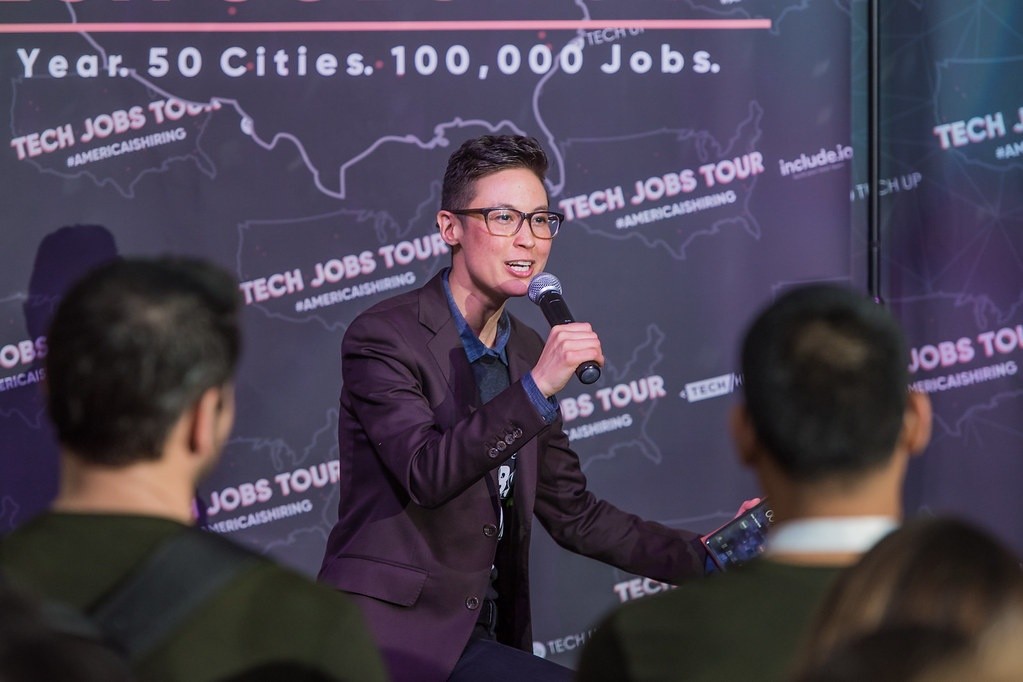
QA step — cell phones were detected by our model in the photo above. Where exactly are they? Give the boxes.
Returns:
[701,498,773,574]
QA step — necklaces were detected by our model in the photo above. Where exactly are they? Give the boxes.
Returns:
[763,515,898,557]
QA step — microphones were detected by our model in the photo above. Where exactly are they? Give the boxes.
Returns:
[528,272,601,385]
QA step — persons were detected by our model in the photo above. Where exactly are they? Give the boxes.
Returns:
[787,511,1023,682]
[0,261,389,682]
[315,133,762,682]
[577,282,931,682]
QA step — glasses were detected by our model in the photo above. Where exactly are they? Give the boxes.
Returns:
[435,207,565,239]
